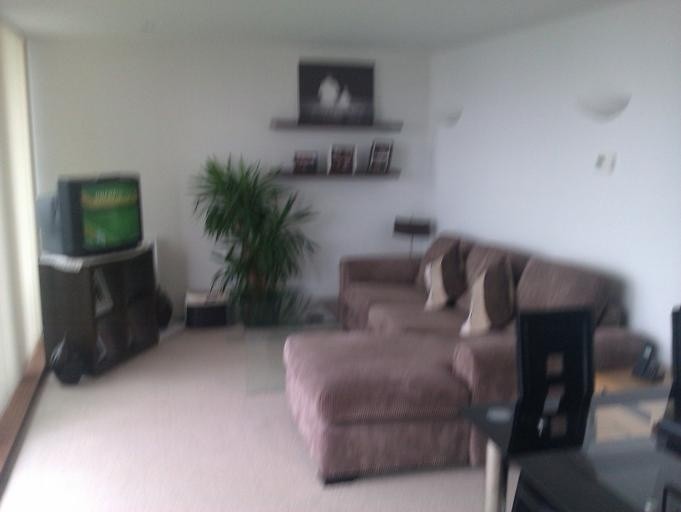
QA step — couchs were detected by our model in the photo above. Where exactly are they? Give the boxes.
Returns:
[341,235,652,470]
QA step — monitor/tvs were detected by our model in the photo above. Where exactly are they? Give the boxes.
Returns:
[35,172,143,255]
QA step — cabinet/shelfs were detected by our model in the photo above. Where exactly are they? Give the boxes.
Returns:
[39,236,161,378]
[269,118,404,179]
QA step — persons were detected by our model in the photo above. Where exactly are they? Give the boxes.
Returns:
[317,72,340,109]
[336,82,352,110]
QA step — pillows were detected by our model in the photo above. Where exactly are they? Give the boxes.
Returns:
[423,239,518,338]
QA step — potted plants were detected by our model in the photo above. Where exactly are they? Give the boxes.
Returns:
[190,147,322,328]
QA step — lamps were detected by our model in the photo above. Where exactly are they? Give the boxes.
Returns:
[393,215,432,258]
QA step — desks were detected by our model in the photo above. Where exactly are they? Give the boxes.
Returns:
[456,385,681,511]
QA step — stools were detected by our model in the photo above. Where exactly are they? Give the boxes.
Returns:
[282,331,473,486]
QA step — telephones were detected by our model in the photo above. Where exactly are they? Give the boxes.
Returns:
[634,342,661,380]
